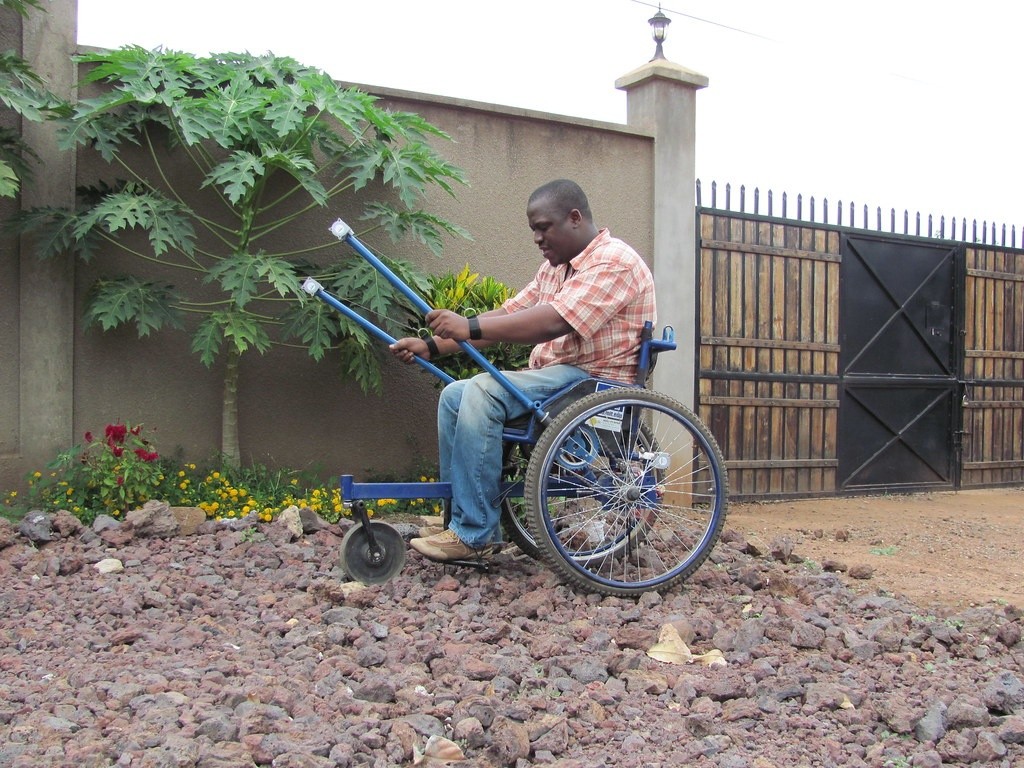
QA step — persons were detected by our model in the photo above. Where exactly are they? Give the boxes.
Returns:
[389,179,658,562]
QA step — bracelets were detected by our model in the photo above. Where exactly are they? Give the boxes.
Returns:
[416,328,441,362]
[464,307,482,342]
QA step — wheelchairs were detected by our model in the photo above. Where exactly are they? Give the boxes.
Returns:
[299,218,731,599]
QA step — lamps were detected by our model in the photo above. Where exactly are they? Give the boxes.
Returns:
[648,3,671,63]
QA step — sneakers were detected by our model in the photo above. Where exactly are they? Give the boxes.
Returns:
[419,526,502,554]
[410,528,493,562]
